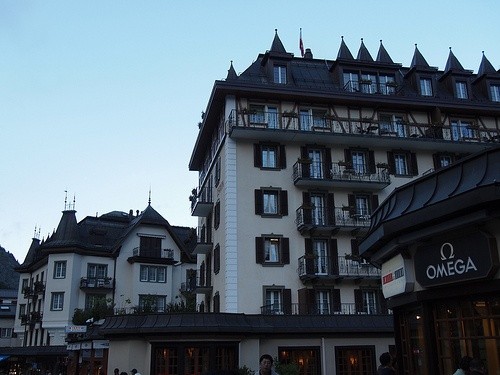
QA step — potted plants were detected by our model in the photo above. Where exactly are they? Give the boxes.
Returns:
[345,252,358,260]
[363,116,374,123]
[385,81,399,87]
[304,251,319,260]
[376,163,386,168]
[187,268,195,279]
[186,228,196,240]
[282,110,298,118]
[338,159,353,166]
[341,204,356,211]
[297,156,313,164]
[432,119,445,125]
[467,123,482,129]
[324,113,336,121]
[396,119,406,125]
[358,78,372,85]
[243,106,257,115]
[301,201,316,210]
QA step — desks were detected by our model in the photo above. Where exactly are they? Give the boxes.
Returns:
[351,213,363,224]
[340,169,353,179]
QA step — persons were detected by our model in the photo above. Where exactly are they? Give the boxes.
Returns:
[254,354,279,375]
[131,369,141,375]
[452,356,488,375]
[114,368,120,375]
[378,349,400,375]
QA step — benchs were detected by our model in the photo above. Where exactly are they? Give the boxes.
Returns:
[310,125,331,132]
[250,121,268,128]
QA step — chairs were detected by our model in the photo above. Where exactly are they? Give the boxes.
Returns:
[330,169,339,178]
